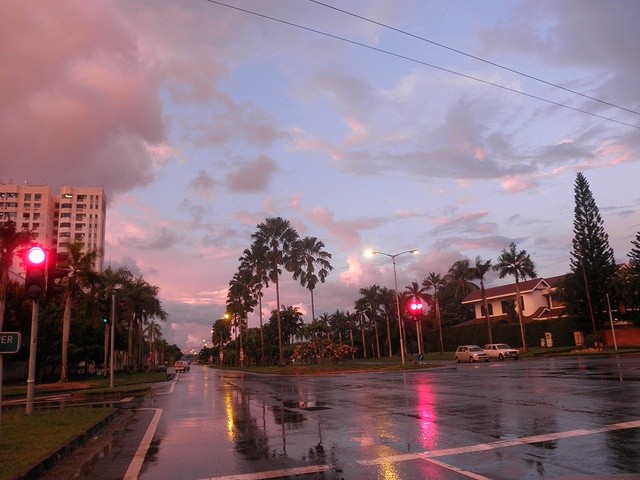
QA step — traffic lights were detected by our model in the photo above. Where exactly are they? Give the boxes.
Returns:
[46,251,70,298]
[98,296,112,324]
[25,242,45,300]
[115,293,127,327]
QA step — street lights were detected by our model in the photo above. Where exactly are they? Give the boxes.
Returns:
[224,315,244,368]
[363,249,420,367]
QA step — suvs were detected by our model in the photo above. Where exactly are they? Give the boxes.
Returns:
[174,361,191,372]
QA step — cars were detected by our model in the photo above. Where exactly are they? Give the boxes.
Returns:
[483,343,520,362]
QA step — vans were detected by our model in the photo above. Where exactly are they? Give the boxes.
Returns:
[455,345,489,363]
